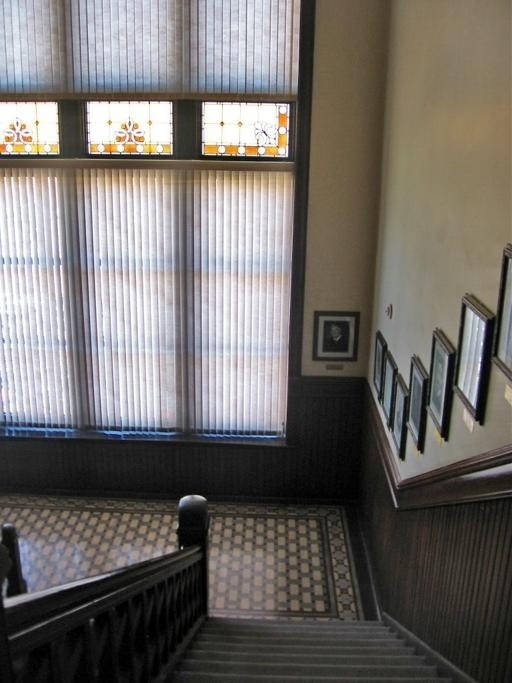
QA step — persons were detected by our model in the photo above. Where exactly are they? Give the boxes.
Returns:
[325,323,347,352]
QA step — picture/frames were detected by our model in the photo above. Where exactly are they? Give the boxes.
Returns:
[370,241,512,460]
[311,310,361,363]
